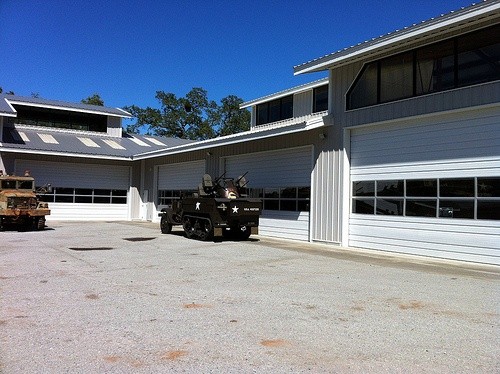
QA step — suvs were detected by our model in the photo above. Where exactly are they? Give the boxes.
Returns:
[0,168,51,230]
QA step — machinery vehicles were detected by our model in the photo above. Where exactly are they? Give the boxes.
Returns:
[157,169,262,242]
